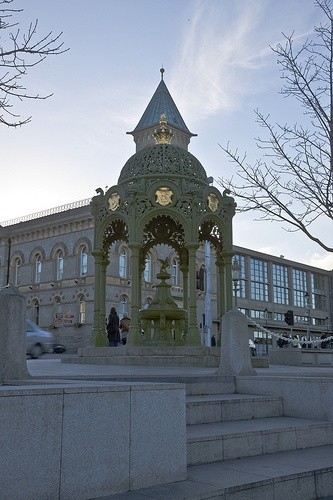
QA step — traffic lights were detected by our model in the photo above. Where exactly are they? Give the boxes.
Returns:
[283,310,292,326]
[196,267,204,292]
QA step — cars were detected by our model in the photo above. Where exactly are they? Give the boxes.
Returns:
[26,319,53,360]
[249,338,257,357]
[47,343,66,355]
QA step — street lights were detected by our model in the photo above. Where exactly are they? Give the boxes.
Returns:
[232,256,242,310]
[263,307,269,355]
[304,292,311,348]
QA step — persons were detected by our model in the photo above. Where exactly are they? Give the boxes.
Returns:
[249,331,333,356]
[105,307,130,347]
[211,333,216,346]
